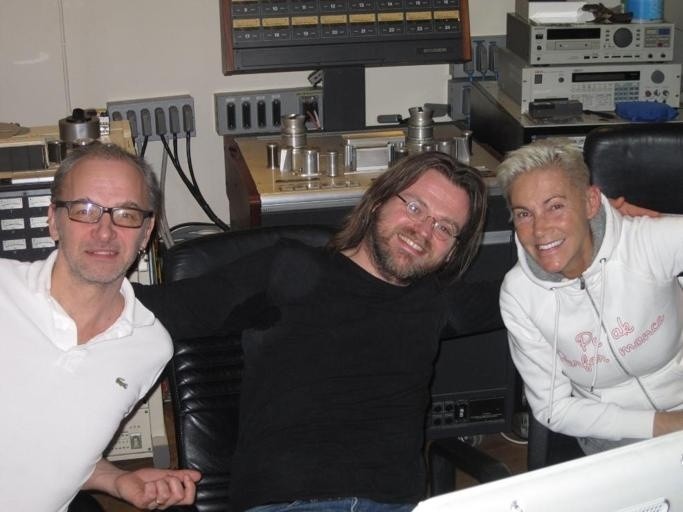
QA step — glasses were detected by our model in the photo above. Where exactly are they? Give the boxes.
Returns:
[396,194,461,241]
[56,200,153,229]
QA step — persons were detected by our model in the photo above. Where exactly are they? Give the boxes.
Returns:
[497,139,683,458]
[131,151,489,511]
[0,139,204,510]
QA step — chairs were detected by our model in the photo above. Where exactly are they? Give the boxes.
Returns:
[163,222,431,512]
[527,122,683,471]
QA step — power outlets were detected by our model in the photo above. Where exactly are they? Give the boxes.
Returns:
[450,35,508,78]
[107,94,197,142]
[212,87,323,135]
[448,81,474,120]
